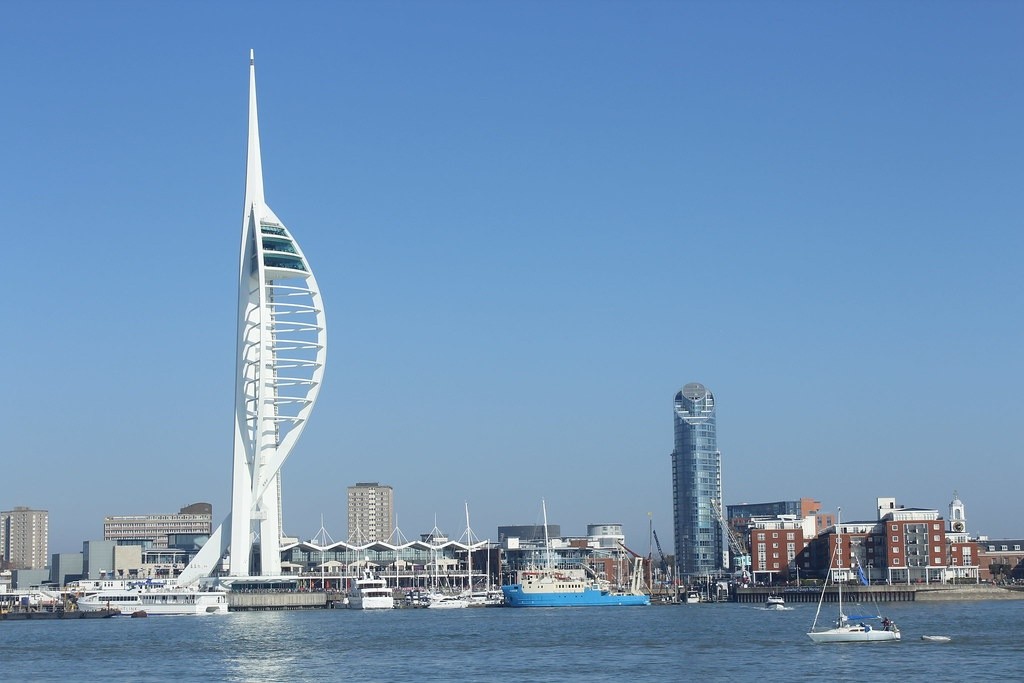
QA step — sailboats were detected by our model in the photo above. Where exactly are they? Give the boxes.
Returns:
[806,505,904,642]
[500,500,651,607]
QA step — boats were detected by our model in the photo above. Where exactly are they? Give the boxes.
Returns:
[324,558,501,609]
[766,596,786,606]
[75,584,230,617]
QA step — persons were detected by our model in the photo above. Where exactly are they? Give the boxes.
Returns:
[881,616,891,630]
